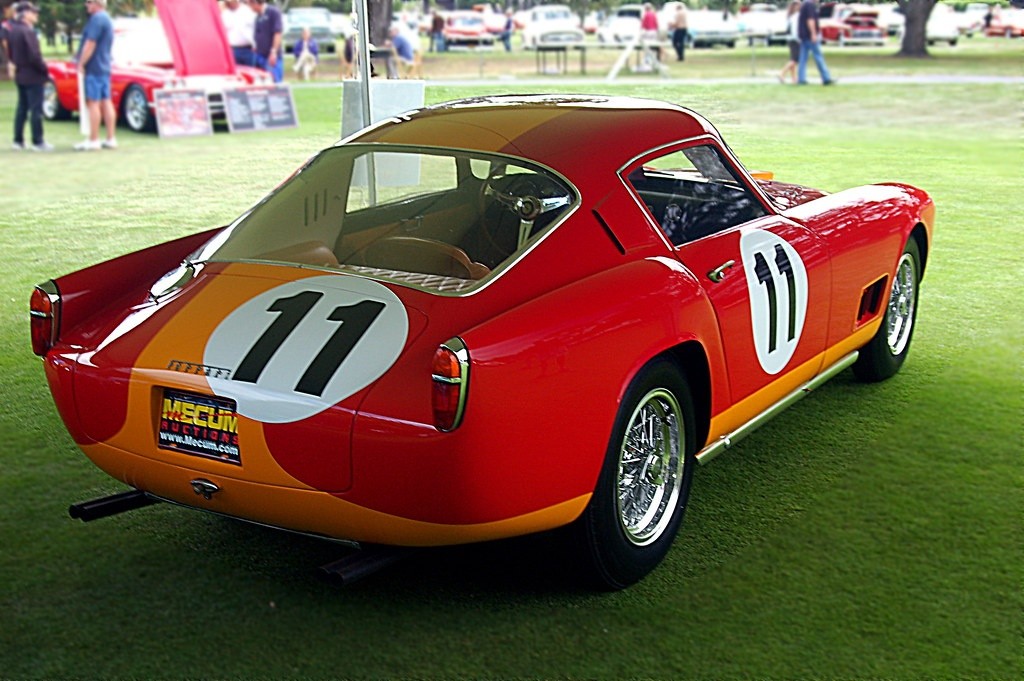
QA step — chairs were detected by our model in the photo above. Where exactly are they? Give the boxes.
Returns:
[337,51,424,82]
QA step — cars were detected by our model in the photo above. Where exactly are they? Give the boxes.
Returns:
[740,2,907,47]
[685,10,740,49]
[600,5,667,50]
[923,1,959,47]
[524,5,585,50]
[44,0,275,134]
[284,5,507,56]
[954,3,1024,40]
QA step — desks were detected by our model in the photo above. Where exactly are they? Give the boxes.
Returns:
[536,43,661,73]
[368,47,393,79]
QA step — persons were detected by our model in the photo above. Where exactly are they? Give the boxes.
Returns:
[641,4,658,39]
[221,0,284,82]
[0,0,54,152]
[797,0,833,85]
[345,37,378,77]
[293,28,318,80]
[502,10,512,51]
[429,10,444,51]
[385,27,414,79]
[669,5,688,60]
[779,0,802,83]
[73,0,117,149]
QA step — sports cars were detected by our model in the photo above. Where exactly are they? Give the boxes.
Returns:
[29,93,937,594]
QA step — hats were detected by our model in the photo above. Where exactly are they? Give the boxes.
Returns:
[15,2,41,14]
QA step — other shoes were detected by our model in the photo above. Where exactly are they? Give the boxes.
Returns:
[73,138,101,150]
[11,142,24,151]
[33,142,52,152]
[100,139,116,151]
